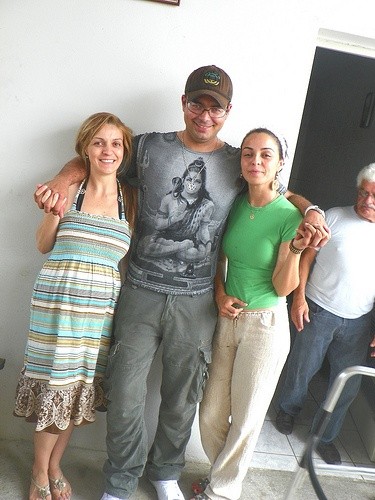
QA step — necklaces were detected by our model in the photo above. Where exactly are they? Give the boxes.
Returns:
[247,190,277,220]
[181,131,219,191]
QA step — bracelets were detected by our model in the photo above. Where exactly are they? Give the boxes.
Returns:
[289,238,303,254]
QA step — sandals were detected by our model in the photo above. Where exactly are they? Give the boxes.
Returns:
[28,470,51,500]
[48,471,72,500]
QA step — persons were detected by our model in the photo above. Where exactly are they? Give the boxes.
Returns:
[190,125,331,500]
[13,112,139,500]
[274,161,375,464]
[34,66,326,500]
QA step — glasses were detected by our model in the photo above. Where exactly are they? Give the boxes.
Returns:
[358,189,375,204]
[187,99,226,119]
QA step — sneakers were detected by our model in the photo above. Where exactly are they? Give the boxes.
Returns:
[99,492,125,500]
[147,477,186,499]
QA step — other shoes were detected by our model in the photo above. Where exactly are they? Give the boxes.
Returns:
[275,409,295,435]
[315,440,342,465]
[188,475,215,500]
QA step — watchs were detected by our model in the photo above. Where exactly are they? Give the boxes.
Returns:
[304,205,325,219]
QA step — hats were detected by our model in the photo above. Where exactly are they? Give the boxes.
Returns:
[185,64,234,110]
